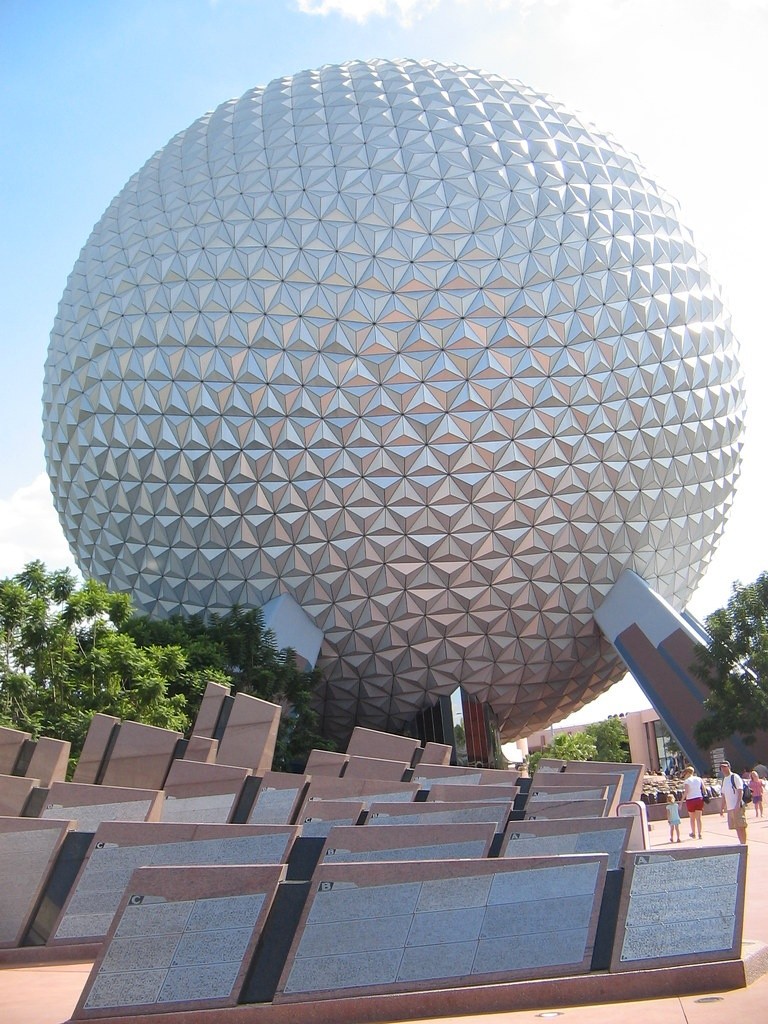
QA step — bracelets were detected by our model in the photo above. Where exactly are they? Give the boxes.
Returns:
[721,807,724,809]
[681,800,684,803]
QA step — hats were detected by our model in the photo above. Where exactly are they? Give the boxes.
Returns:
[719,760,730,767]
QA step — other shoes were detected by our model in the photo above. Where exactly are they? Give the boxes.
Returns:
[688,832,696,838]
[698,835,704,838]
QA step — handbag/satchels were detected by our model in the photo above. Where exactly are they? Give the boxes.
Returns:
[731,772,754,803]
[701,790,711,804]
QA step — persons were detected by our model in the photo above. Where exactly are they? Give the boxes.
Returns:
[660,766,675,775]
[666,794,683,843]
[742,760,768,779]
[720,761,750,855]
[748,771,766,818]
[679,767,706,840]
[686,763,694,772]
